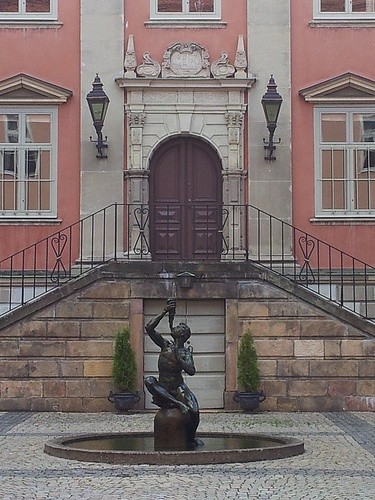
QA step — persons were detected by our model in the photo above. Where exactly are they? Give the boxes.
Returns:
[145,297,204,447]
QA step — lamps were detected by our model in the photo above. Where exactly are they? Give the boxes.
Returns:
[261,74,283,160]
[86,73,110,159]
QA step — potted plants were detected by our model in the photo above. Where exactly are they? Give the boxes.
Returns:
[108,328,141,414]
[232,328,266,414]
